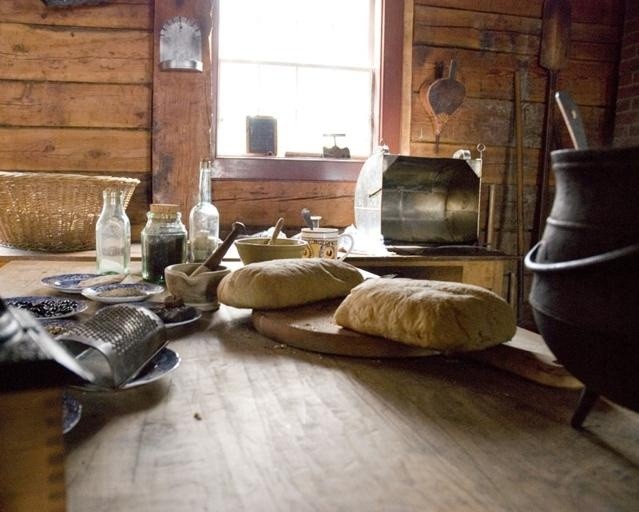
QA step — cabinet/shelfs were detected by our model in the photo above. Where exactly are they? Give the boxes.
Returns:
[343,250,521,296]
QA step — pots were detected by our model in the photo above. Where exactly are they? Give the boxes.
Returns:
[523,146,639,430]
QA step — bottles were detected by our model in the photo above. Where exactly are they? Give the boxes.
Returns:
[95,191,131,276]
[188,157,222,262]
[139,201,189,284]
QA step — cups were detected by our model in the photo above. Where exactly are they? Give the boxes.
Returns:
[300,226,354,263]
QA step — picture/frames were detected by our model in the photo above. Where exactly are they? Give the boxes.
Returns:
[244,115,281,157]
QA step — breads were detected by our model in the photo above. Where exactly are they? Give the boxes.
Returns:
[334,278,517,350]
[217,258,364,309]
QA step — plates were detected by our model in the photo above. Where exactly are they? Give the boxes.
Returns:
[1,296,89,321]
[33,320,182,435]
[40,273,115,293]
[94,301,204,328]
[80,282,165,304]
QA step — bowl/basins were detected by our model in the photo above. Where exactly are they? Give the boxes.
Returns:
[164,262,231,311]
[233,237,308,268]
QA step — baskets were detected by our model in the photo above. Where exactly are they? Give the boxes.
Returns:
[0,170,140,253]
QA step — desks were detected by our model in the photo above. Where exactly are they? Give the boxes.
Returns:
[0,258,637,511]
